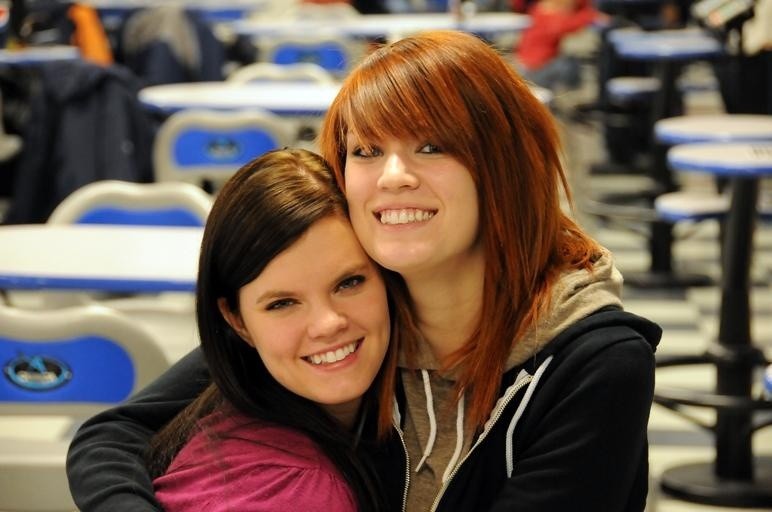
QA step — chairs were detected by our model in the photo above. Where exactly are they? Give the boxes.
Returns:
[1,305,175,510]
[43,177,212,225]
[150,103,295,194]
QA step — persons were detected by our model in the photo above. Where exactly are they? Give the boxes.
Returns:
[63,29,663,511]
[0,0,609,95]
[138,145,425,512]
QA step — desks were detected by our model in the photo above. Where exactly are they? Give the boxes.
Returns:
[137,76,337,156]
[0,220,208,293]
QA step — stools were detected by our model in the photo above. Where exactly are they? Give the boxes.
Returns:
[575,11,772,509]
